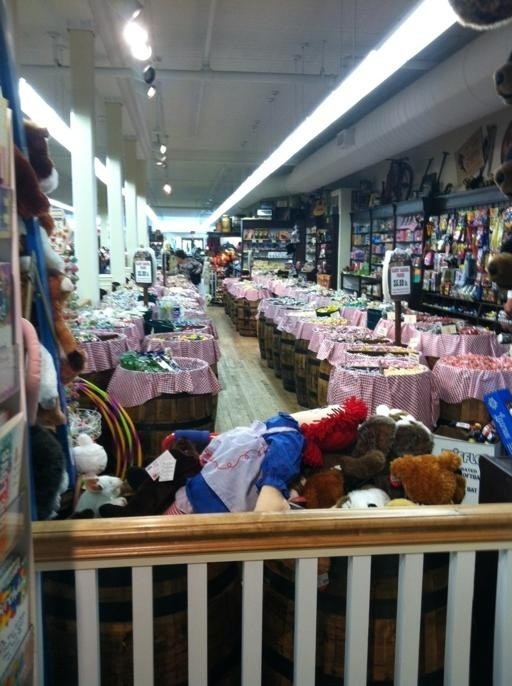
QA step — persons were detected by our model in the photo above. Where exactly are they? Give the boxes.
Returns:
[161,251,207,310]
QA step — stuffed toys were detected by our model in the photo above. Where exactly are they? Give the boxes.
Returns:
[13,123,84,429]
[69,398,467,614]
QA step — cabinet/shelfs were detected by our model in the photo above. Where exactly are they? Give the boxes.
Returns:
[420,184,511,336]
[341,198,438,311]
[241,217,335,287]
